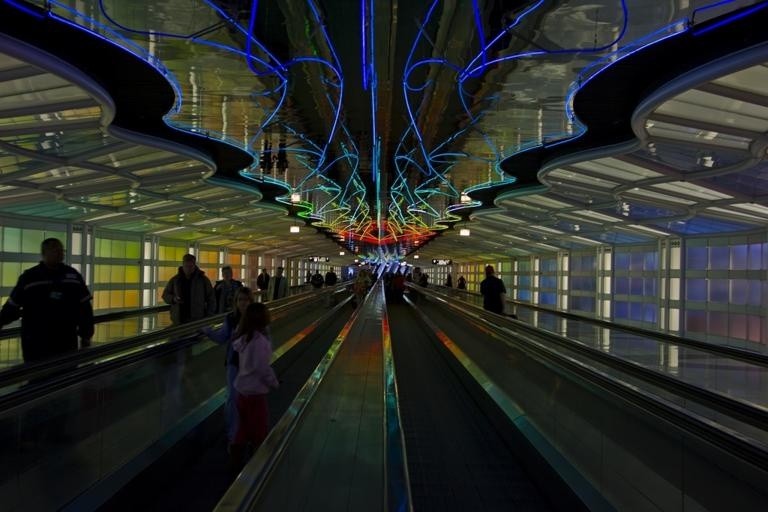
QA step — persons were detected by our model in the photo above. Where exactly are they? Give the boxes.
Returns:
[161,255,216,323]
[446,273,451,287]
[324,267,336,288]
[0,237,95,448]
[256,268,270,301]
[260,138,291,176]
[310,269,323,289]
[212,266,242,313]
[479,266,506,314]
[457,275,466,288]
[353,269,429,303]
[151,343,192,429]
[305,271,311,281]
[230,302,279,448]
[266,267,288,301]
[201,287,274,437]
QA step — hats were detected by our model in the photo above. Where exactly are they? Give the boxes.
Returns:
[486,265,494,274]
